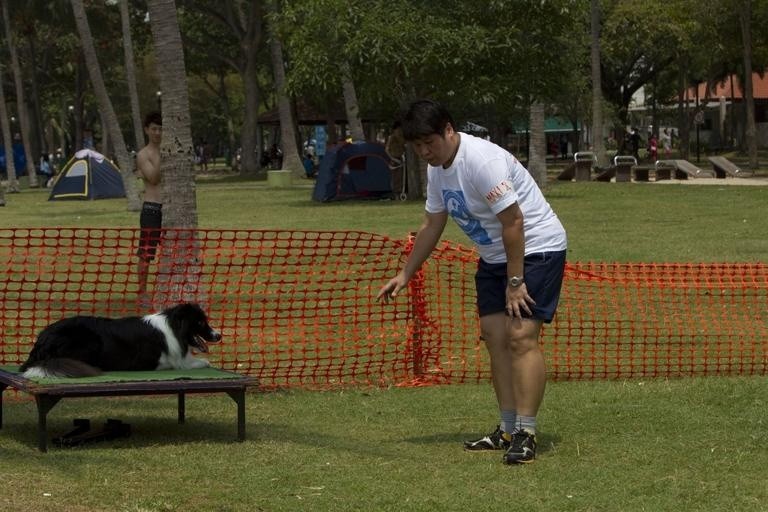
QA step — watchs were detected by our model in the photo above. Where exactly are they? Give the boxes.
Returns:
[506,276,524,287]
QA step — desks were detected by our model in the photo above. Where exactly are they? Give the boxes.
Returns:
[1,362,257,454]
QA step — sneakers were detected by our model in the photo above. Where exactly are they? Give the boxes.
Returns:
[500,426,538,466]
[462,423,512,454]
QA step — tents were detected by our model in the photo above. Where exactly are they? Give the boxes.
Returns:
[313,139,405,203]
[47,148,128,200]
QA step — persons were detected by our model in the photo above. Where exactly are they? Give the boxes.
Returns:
[231,144,284,171]
[303,154,316,178]
[626,129,645,164]
[304,135,317,155]
[560,135,567,160]
[560,141,560,163]
[663,137,671,160]
[374,99,568,465]
[40,152,54,186]
[136,112,162,303]
[196,145,208,174]
[647,134,658,163]
[663,129,672,148]
[385,124,407,201]
[677,129,690,160]
[53,148,66,175]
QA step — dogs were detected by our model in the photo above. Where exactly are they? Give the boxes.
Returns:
[18,303,222,378]
